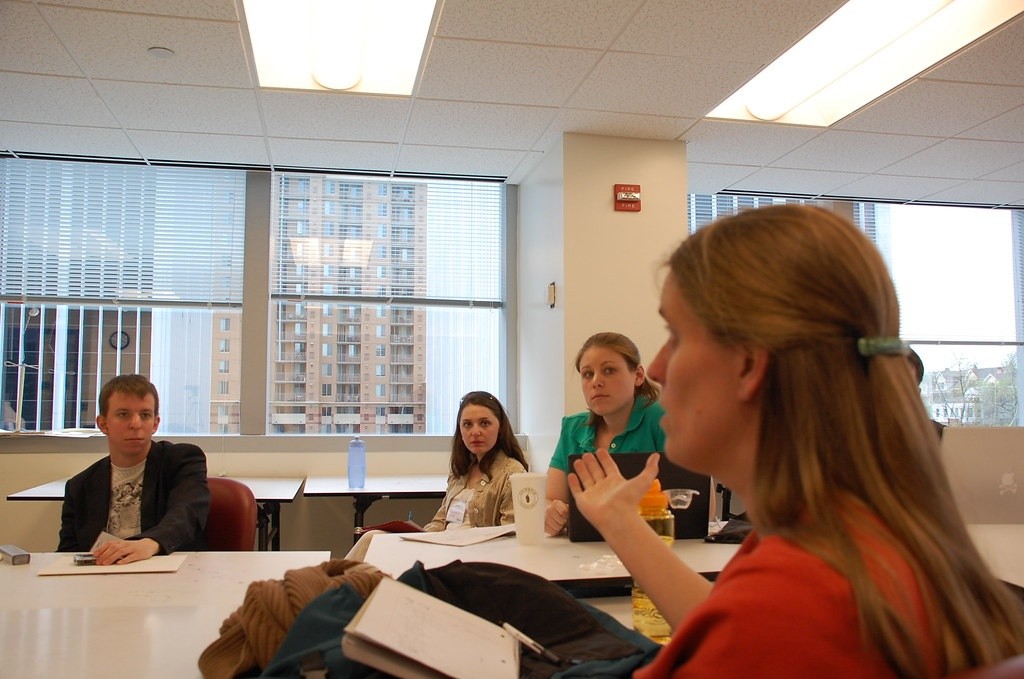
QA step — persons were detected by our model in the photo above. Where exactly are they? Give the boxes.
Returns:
[567,203,1024,679]
[423,391,529,532]
[545,332,716,536]
[57,373,210,566]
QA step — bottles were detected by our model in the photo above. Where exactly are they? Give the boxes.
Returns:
[347,436,367,489]
[631,478,677,646]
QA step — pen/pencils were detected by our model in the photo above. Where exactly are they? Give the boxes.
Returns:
[497,620,562,666]
[408,511,412,521]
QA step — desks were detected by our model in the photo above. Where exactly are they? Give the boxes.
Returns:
[0,550,333,679]
[303,473,461,549]
[7,475,303,551]
[362,534,745,630]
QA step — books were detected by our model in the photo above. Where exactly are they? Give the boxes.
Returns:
[342,576,521,679]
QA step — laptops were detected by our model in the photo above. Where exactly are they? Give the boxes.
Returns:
[567,453,710,543]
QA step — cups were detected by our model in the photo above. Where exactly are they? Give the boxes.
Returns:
[509,473,548,545]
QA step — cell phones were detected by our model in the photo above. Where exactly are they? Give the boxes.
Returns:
[74,553,125,566]
